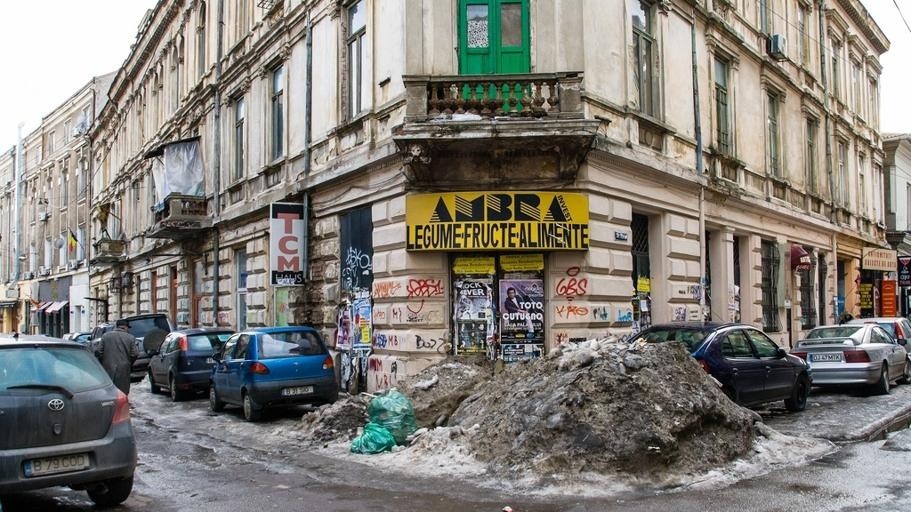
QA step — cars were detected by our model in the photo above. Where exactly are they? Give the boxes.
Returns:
[206,327,339,420]
[627,322,813,412]
[789,323,910,393]
[848,316,911,353]
[144,328,239,403]
[0,331,138,510]
[69,331,91,344]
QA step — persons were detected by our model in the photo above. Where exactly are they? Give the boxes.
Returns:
[505,287,528,326]
[98,318,140,398]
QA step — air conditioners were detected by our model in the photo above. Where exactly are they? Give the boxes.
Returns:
[770,32,791,64]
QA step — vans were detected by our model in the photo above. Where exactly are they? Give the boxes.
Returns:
[112,314,174,380]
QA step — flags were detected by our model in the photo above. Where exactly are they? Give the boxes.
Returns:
[68,228,78,257]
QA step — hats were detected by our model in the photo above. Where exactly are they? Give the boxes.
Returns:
[117,319,132,328]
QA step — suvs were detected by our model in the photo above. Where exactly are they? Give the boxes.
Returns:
[87,322,115,358]
